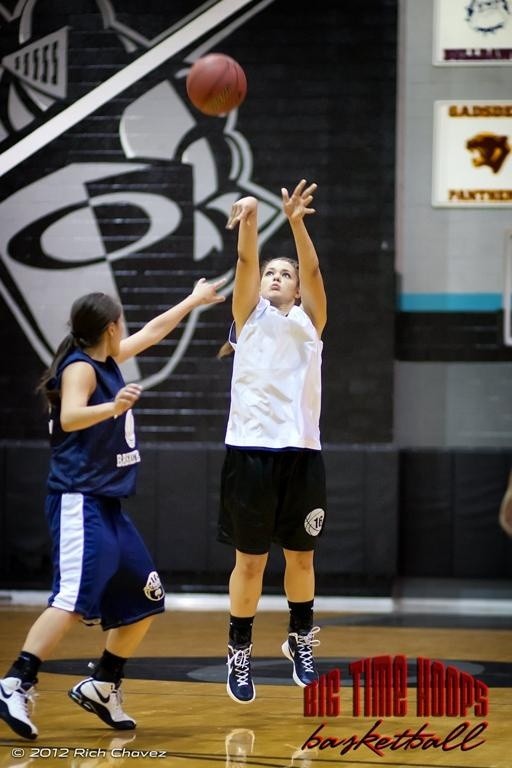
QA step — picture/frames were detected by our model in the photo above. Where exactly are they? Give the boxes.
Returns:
[430,0,511,210]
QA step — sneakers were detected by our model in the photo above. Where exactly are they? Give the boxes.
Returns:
[287,749,318,768]
[225,729,255,768]
[226,640,256,704]
[282,626,320,688]
[68,677,136,730]
[0,677,39,739]
[72,732,136,768]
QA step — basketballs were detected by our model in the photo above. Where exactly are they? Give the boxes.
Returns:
[187,52,248,117]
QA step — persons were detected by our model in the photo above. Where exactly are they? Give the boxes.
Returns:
[213,175,329,706]
[0,272,230,744]
